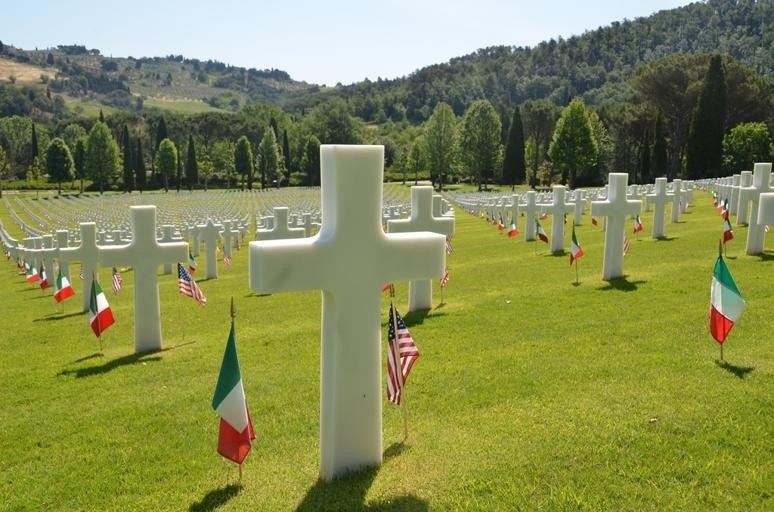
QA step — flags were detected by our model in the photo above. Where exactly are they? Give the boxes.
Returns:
[6,251,123,339]
[386,303,420,407]
[177,262,206,307]
[708,255,747,345]
[712,191,735,244]
[186,240,232,275]
[439,210,644,291]
[209,321,257,465]
